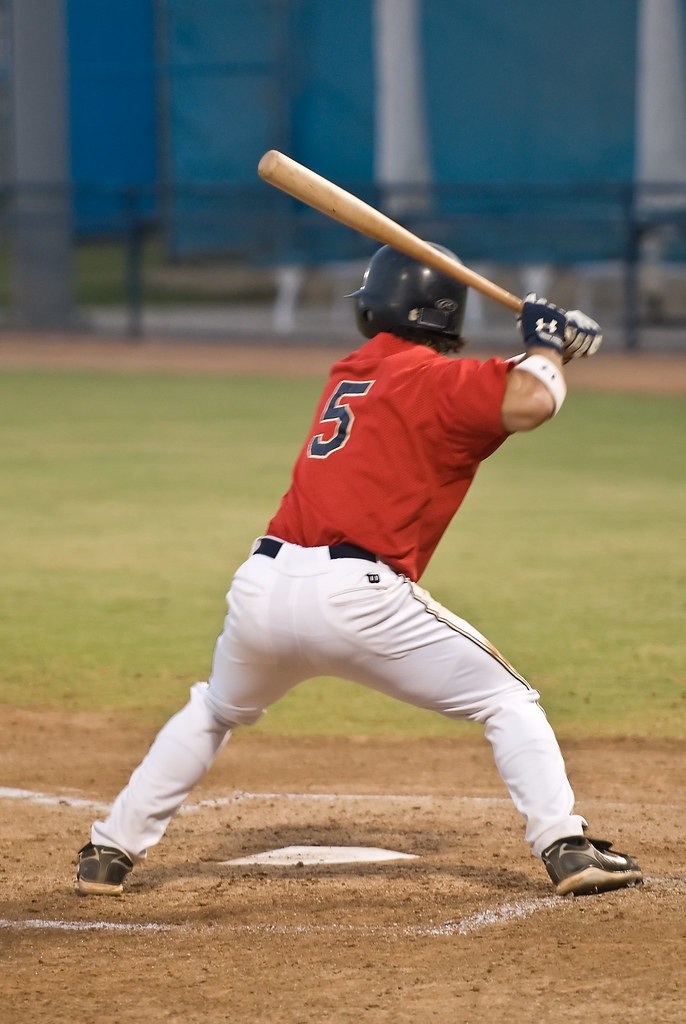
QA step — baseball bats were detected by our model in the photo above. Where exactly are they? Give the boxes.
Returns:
[256,149,525,312]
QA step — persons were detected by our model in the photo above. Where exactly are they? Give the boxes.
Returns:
[74,241,644,896]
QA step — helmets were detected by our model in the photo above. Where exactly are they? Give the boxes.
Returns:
[344,238,467,338]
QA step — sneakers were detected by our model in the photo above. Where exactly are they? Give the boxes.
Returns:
[76,842,134,895]
[540,835,643,895]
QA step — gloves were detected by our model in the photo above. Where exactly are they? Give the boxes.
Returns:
[562,309,602,366]
[515,291,566,357]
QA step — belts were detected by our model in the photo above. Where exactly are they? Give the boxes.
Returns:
[255,536,381,563]
